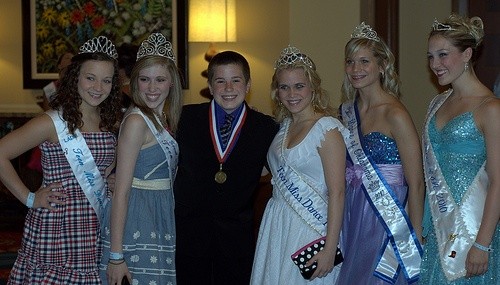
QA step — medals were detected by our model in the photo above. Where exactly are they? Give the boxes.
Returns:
[215,172,226,183]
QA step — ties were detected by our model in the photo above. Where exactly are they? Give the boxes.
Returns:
[219,114,235,148]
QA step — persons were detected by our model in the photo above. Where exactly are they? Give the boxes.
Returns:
[108,52,282,285]
[336,21,426,285]
[105,33,180,285]
[419,14,500,285]
[249,43,344,285]
[0,36,117,285]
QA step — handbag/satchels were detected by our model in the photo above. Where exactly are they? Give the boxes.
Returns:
[291,234,345,280]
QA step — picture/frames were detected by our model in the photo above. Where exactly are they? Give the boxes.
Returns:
[21,0,190,92]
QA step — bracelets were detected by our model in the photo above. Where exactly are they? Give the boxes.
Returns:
[26,191,35,209]
[107,258,125,265]
[108,251,124,260]
[473,241,492,253]
